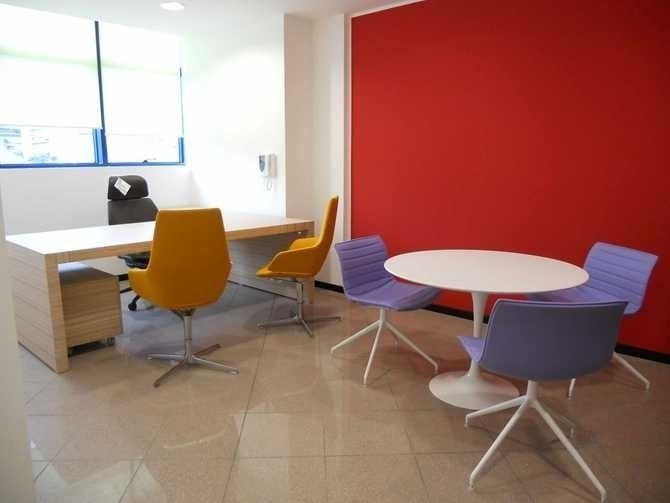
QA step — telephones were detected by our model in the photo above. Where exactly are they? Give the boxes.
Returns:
[258,153,277,177]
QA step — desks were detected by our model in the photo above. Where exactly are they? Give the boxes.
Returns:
[382,249,589,411]
[5,213,316,373]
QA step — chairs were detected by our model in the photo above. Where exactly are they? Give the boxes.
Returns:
[331,235,442,386]
[529,241,659,400]
[107,174,159,310]
[127,209,238,388]
[255,195,341,337]
[455,299,629,503]
